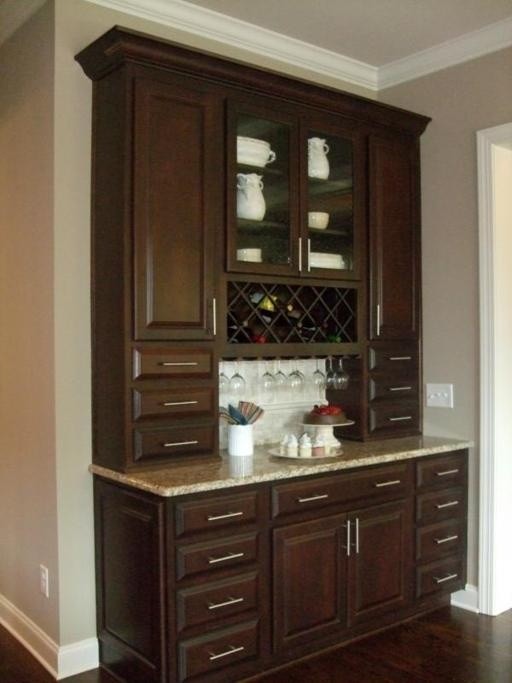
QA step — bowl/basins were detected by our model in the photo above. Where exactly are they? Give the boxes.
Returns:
[308,211,331,230]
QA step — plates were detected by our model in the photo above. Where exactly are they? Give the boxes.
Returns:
[289,420,358,447]
[267,447,346,461]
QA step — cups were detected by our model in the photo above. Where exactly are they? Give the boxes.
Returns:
[236,247,264,263]
[271,359,288,391]
[324,355,343,389]
[217,361,232,395]
[259,361,277,392]
[310,357,325,390]
[285,358,304,393]
[332,356,350,392]
[229,361,247,398]
[229,423,254,458]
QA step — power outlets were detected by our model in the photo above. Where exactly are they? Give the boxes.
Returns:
[39,563,49,600]
[426,383,454,409]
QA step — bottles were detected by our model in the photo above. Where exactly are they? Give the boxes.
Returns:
[250,293,293,314]
[230,329,265,344]
[255,311,302,328]
[324,335,342,343]
[296,320,329,337]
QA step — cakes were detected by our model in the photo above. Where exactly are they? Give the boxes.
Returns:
[287,434,298,456]
[312,436,325,456]
[305,405,347,426]
[280,436,288,453]
[299,432,312,456]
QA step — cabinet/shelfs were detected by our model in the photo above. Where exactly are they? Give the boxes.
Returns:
[86,436,476,683]
[74,26,431,471]
